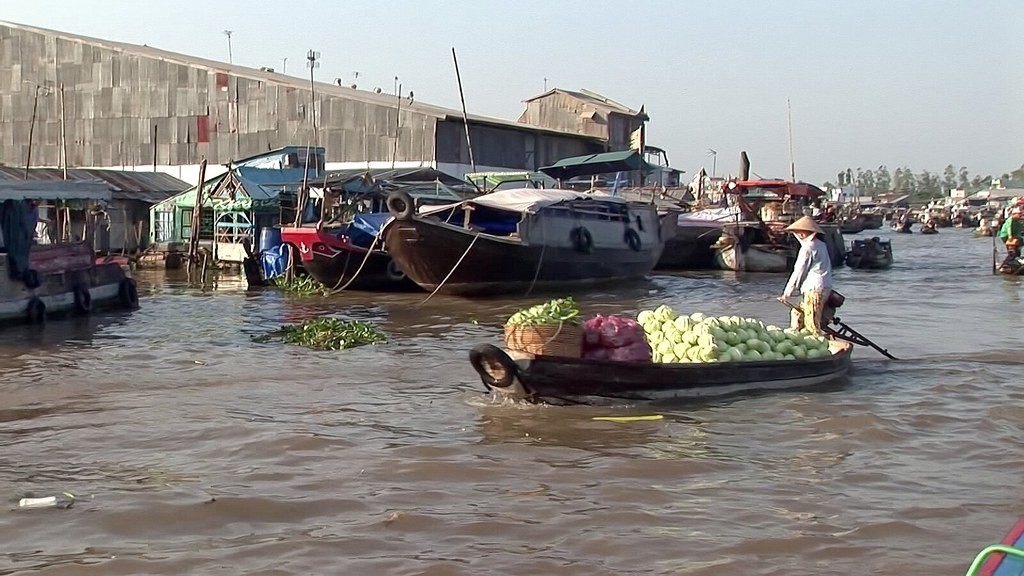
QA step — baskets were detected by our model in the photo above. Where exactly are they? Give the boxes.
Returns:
[504,324,583,360]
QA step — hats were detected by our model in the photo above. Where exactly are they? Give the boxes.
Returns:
[1011,208,1022,214]
[784,215,826,234]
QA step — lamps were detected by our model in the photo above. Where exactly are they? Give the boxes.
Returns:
[407,91,414,101]
[373,87,382,94]
[260,65,274,72]
[350,84,357,89]
[333,77,341,87]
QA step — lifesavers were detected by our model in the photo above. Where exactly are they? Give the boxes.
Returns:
[119,278,138,308]
[469,341,517,388]
[72,285,93,312]
[576,226,594,254]
[27,296,46,315]
[387,192,417,217]
[625,228,641,251]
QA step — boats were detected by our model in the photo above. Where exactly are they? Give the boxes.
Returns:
[468,289,853,407]
[278,47,1024,296]
[0,178,136,322]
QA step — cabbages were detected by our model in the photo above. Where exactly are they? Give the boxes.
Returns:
[506,300,576,324]
[637,304,830,364]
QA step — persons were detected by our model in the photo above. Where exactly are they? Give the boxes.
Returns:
[781,215,833,338]
[887,196,1024,267]
[33,200,113,246]
